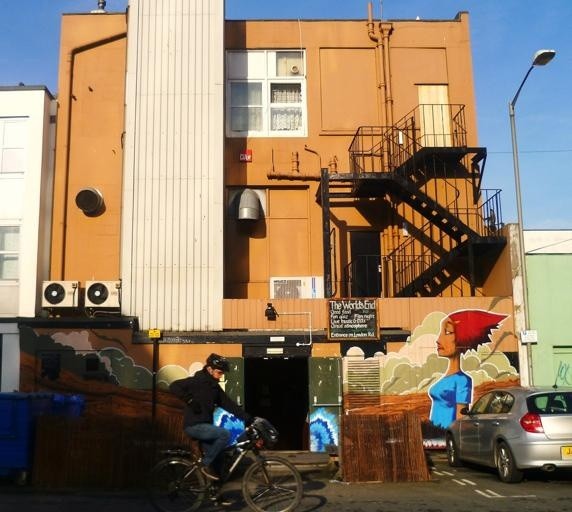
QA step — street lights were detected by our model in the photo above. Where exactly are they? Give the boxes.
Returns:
[510,45,560,386]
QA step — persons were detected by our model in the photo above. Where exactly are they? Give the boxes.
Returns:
[183,353,256,505]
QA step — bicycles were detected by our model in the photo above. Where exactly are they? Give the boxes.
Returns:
[144,417,305,511]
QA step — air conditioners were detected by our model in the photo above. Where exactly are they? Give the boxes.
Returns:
[40,279,121,311]
[269,276,325,300]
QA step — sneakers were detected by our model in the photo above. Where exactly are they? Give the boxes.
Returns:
[202,466,220,481]
[221,498,235,506]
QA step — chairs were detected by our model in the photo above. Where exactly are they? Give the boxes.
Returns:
[547,400,568,412]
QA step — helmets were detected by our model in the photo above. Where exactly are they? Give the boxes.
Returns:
[206,352,231,374]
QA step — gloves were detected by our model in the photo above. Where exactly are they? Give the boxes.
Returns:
[187,399,202,414]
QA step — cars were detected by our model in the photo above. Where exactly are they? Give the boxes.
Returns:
[445,383,572,484]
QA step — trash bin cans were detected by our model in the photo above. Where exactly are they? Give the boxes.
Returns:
[0,391,85,484]
